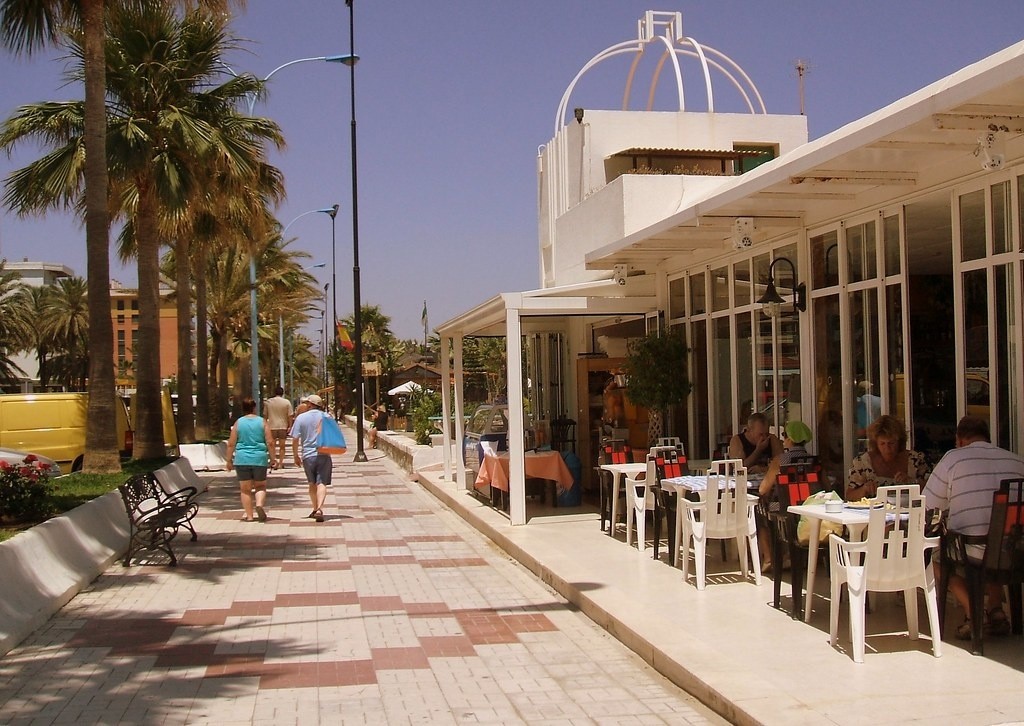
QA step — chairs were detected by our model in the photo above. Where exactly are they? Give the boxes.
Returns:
[656,437,686,457]
[938,477,1024,656]
[697,442,732,547]
[649,449,728,568]
[681,458,762,590]
[624,442,685,552]
[776,472,872,620]
[593,436,645,537]
[759,454,824,574]
[829,485,942,662]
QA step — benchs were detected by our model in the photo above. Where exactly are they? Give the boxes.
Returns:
[118,471,199,568]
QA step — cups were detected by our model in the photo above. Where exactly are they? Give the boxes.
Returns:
[824,501,843,513]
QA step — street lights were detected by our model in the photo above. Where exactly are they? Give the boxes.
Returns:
[278,208,332,397]
[248,53,359,412]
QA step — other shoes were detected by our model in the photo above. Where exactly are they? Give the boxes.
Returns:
[783,559,791,569]
[761,563,771,573]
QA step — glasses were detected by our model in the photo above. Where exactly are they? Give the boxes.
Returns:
[781,432,786,436]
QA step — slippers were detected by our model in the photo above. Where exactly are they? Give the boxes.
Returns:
[240,517,254,522]
[255,506,267,523]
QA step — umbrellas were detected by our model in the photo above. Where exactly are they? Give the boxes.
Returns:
[388,380,434,399]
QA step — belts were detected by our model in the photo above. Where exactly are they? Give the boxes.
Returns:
[961,534,987,544]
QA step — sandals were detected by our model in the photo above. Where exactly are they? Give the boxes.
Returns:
[308,511,316,518]
[983,607,1010,635]
[315,508,324,522]
[954,615,972,640]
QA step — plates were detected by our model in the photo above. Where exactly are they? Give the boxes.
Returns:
[843,503,884,509]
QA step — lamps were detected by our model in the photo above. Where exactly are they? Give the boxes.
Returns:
[574,108,584,123]
[756,257,806,316]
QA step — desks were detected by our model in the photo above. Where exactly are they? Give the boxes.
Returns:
[660,475,762,578]
[474,448,576,512]
[787,490,927,644]
[600,460,712,546]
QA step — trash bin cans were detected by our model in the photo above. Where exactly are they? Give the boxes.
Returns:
[557,449,581,509]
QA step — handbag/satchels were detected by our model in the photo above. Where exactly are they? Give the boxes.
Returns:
[316,412,346,454]
[796,490,843,547]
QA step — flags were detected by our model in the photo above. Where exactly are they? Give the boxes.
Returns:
[421,307,429,325]
[337,322,353,349]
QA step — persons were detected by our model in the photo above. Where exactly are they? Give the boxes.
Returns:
[729,413,784,475]
[846,415,931,501]
[919,416,1024,640]
[756,422,815,572]
[263,387,293,470]
[288,395,332,522]
[294,396,308,417]
[856,380,874,424]
[364,404,387,450]
[226,396,278,522]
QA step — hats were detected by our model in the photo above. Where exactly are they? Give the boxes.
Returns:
[786,420,812,443]
[303,395,322,407]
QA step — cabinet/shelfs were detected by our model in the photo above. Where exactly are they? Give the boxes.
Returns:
[576,356,643,494]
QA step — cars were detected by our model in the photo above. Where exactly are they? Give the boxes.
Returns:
[1,390,197,479]
[764,358,991,440]
